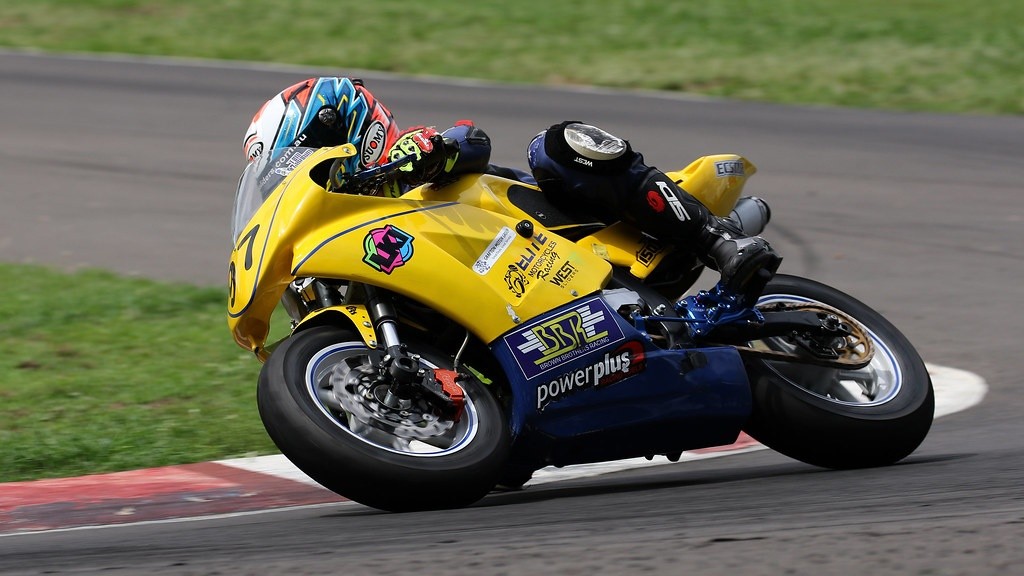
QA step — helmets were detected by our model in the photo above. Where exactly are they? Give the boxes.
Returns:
[242,76,401,198]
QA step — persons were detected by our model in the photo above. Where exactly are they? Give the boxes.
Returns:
[242,76,778,297]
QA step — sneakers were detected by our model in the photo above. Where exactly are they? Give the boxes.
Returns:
[692,215,779,292]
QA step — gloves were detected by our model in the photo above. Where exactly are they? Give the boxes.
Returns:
[385,125,447,180]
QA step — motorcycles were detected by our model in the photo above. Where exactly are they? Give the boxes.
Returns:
[226,139,937,510]
